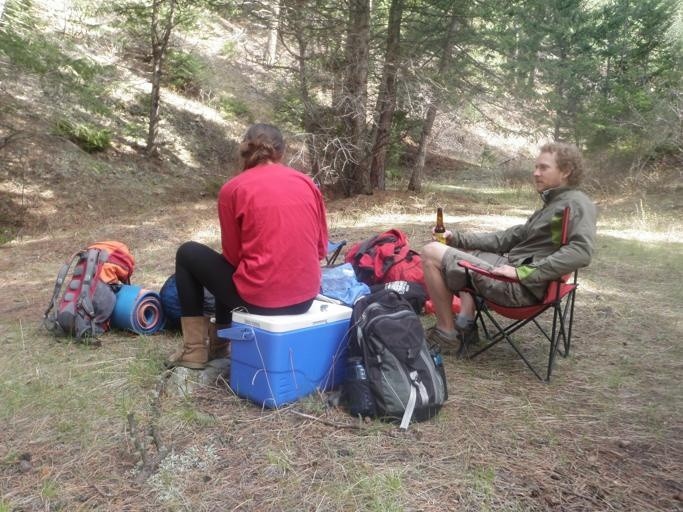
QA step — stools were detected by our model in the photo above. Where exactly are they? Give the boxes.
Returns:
[323,239,347,265]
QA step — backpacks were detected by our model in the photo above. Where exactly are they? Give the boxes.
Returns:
[345,290,449,422]
[45,238,135,343]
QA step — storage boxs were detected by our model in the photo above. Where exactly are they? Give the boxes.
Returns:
[216,298,353,410]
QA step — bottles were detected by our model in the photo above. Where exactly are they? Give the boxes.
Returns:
[345,359,374,417]
[431,350,449,401]
[434,206,447,247]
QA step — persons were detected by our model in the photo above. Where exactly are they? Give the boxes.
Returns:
[164,123,331,371]
[419,141,597,356]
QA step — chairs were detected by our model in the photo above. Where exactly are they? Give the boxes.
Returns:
[455,207,582,382]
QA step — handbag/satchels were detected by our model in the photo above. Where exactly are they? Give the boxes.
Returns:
[346,227,461,313]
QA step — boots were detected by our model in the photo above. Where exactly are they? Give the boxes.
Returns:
[207,322,230,359]
[164,315,209,370]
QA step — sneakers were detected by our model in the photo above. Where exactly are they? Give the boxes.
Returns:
[425,324,464,355]
[454,320,480,345]
